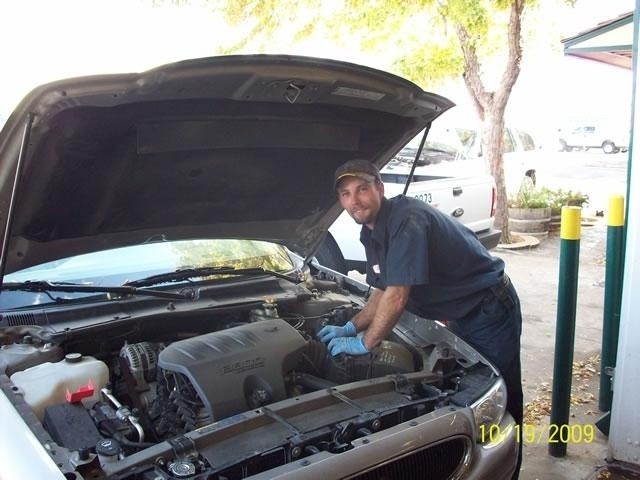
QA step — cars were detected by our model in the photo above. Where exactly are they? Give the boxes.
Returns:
[332,135,501,271]
[555,120,630,155]
[0,53,520,480]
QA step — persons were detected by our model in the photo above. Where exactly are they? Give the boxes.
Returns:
[317,158,524,480]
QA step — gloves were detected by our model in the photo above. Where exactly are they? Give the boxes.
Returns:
[316,321,369,357]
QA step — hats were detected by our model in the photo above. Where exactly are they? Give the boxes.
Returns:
[334,160,381,186]
